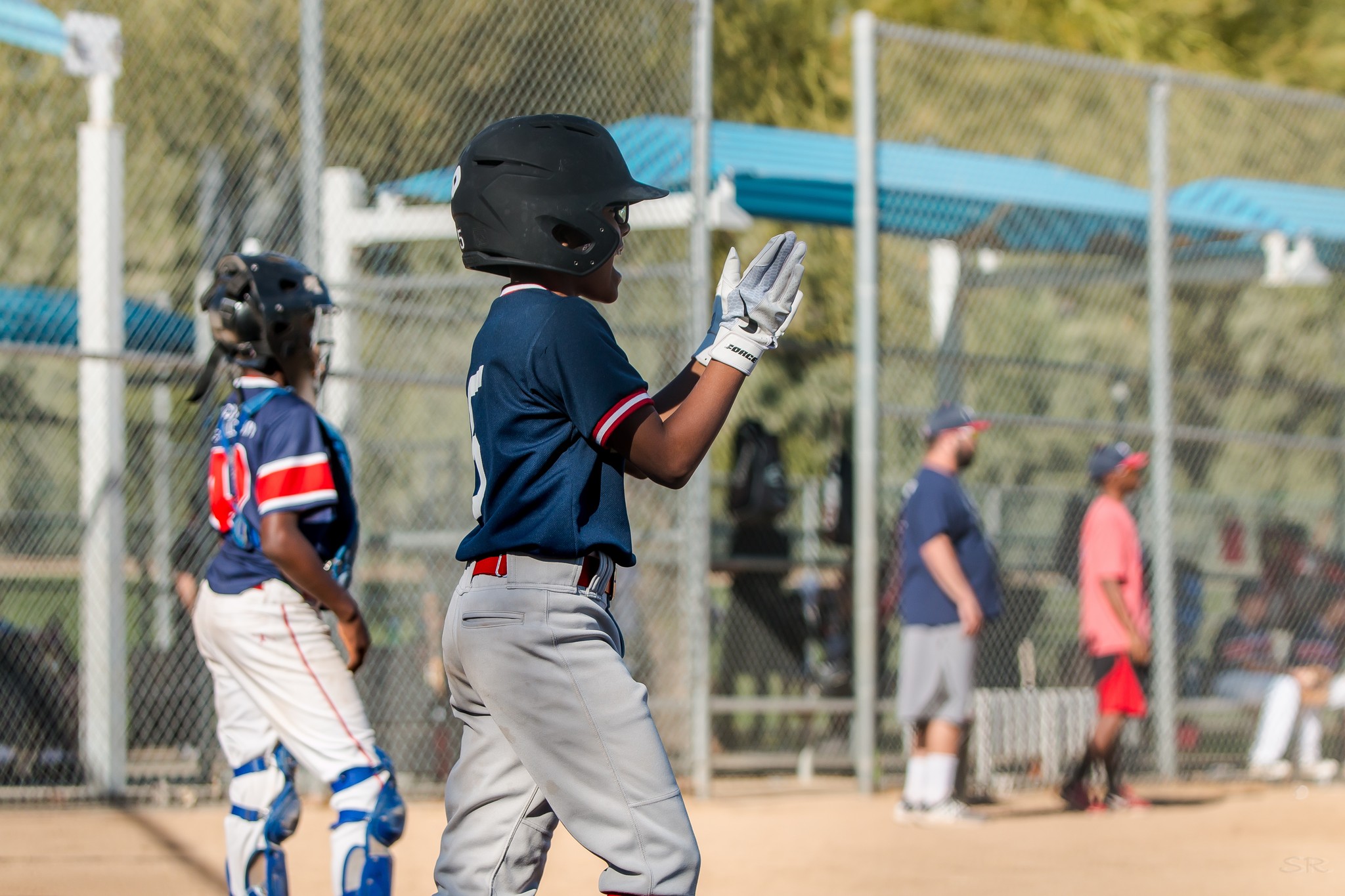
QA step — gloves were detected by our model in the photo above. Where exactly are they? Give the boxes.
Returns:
[691,229,807,377]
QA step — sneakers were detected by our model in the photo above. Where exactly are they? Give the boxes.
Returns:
[1103,789,1148,811]
[1059,780,1111,810]
[894,793,992,829]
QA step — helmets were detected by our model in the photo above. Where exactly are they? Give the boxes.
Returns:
[450,113,670,279]
[199,253,339,377]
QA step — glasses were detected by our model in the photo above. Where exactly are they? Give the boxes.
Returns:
[605,201,629,224]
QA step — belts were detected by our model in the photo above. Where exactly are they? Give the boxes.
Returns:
[472,552,616,601]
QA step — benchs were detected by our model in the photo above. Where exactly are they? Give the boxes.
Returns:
[1175,697,1345,762]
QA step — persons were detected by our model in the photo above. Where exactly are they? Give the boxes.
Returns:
[191,253,405,895]
[900,400,1002,820]
[421,114,806,896]
[1145,521,1345,783]
[176,513,215,784]
[1058,437,1149,810]
[725,407,891,737]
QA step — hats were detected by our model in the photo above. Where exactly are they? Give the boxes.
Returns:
[917,404,996,446]
[1086,439,1150,487]
